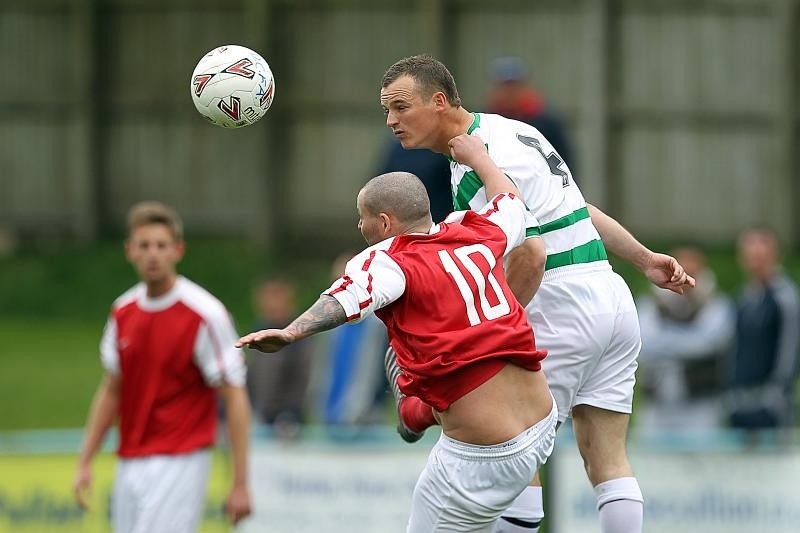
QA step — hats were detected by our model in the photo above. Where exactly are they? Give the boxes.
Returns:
[491,50,527,83]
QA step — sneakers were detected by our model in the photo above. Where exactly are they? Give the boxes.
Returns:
[383,345,426,444]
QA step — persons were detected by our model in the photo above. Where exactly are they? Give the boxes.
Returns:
[377,54,698,533]
[233,132,561,533]
[323,241,391,434]
[73,195,255,532]
[717,228,799,448]
[630,237,737,443]
[211,272,310,426]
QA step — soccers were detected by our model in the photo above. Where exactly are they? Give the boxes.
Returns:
[190,43,278,130]
